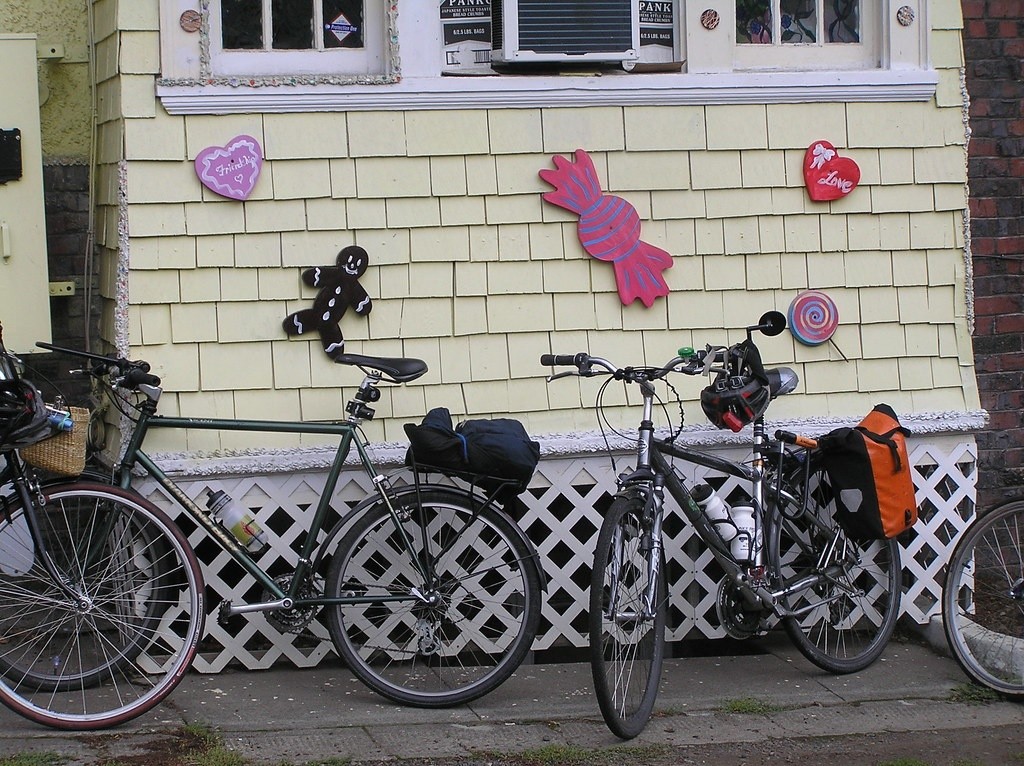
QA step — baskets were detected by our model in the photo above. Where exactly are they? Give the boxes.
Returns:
[19,403,90,475]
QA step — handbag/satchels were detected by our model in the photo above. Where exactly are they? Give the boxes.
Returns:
[819,403,918,540]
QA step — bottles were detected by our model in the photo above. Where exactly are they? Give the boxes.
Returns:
[730,495,755,564]
[205,489,268,553]
[690,484,740,543]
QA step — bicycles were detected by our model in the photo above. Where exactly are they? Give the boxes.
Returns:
[537,308,919,738]
[0,321,207,731]
[0,342,549,713]
[940,499,1024,708]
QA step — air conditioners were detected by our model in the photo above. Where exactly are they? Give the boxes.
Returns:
[492,0,639,65]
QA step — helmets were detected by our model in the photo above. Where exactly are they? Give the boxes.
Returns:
[701,376,770,433]
[0,379,47,444]
